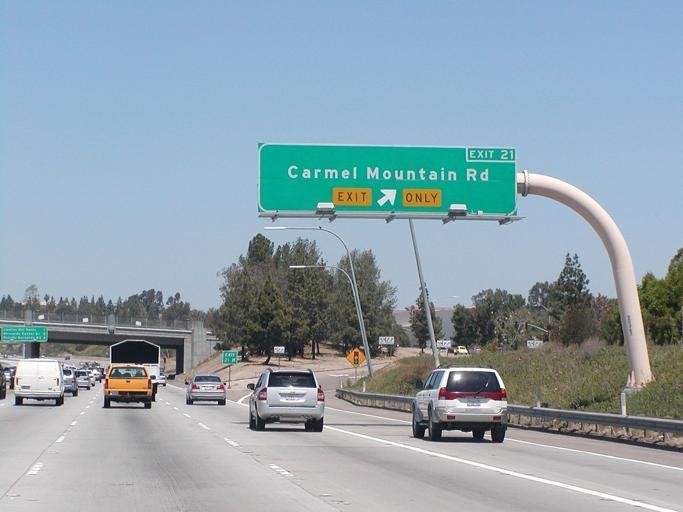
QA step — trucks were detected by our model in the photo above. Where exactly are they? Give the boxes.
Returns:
[108,337,161,369]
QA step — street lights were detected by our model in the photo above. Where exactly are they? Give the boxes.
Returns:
[258,221,380,386]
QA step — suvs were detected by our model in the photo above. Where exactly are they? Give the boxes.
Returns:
[12,357,71,405]
[410,364,509,441]
[184,374,235,412]
[247,368,325,432]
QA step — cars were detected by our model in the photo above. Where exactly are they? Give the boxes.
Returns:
[54,366,80,400]
[102,363,157,409]
[76,363,105,390]
[452,344,471,358]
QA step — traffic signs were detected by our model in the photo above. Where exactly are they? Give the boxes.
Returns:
[257,143,517,224]
[221,351,239,366]
